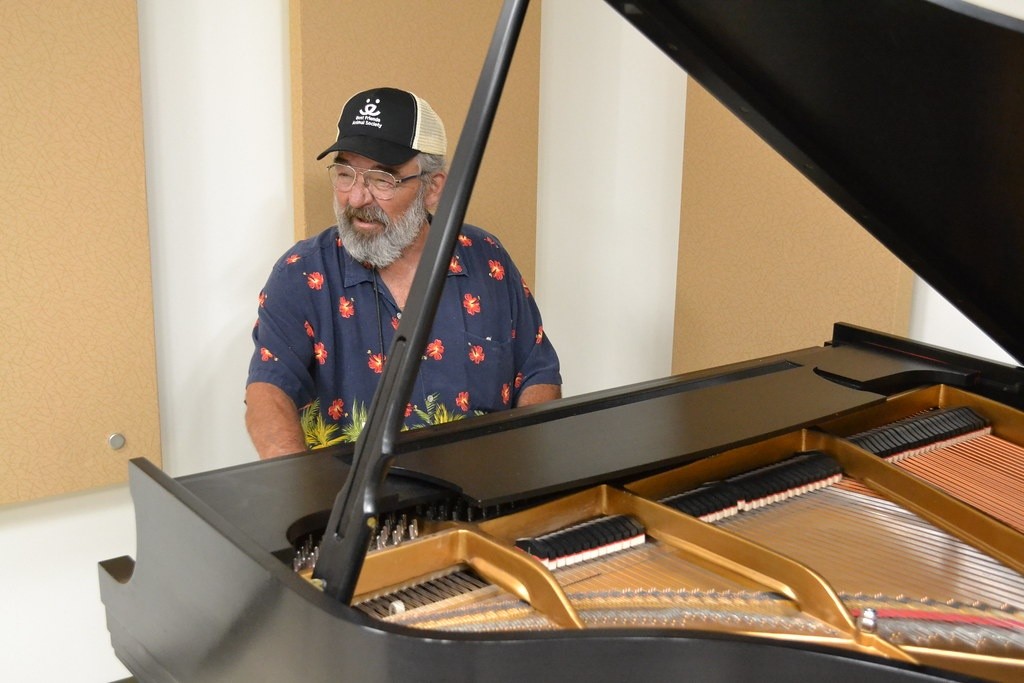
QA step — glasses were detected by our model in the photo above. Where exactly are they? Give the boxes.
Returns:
[327,162,433,200]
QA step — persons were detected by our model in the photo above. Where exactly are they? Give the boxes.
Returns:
[245,87,563,460]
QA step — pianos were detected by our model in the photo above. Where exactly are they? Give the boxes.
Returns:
[95,0,1024,683]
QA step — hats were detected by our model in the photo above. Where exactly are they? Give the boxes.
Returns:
[316,87,446,166]
